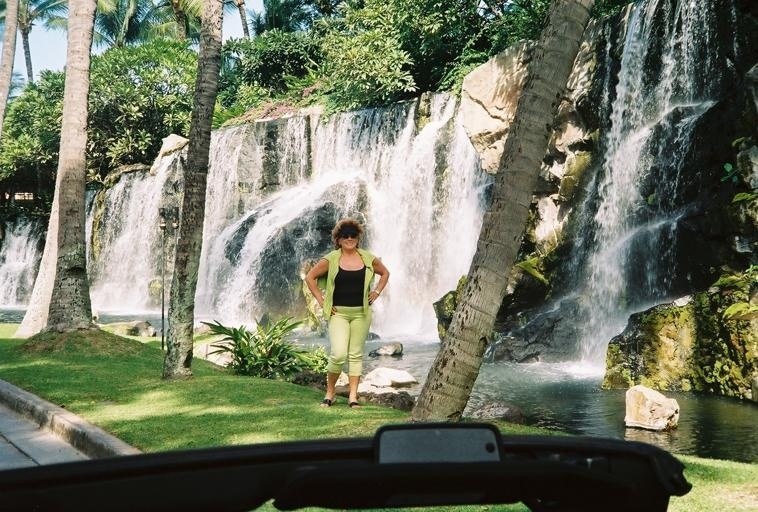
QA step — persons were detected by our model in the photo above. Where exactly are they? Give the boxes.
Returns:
[304,220,390,408]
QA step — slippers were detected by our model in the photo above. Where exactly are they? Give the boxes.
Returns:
[320,397,336,408]
[349,401,360,408]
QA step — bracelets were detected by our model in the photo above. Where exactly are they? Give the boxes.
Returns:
[374,287,381,295]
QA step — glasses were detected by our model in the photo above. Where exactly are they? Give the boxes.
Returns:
[341,233,358,238]
